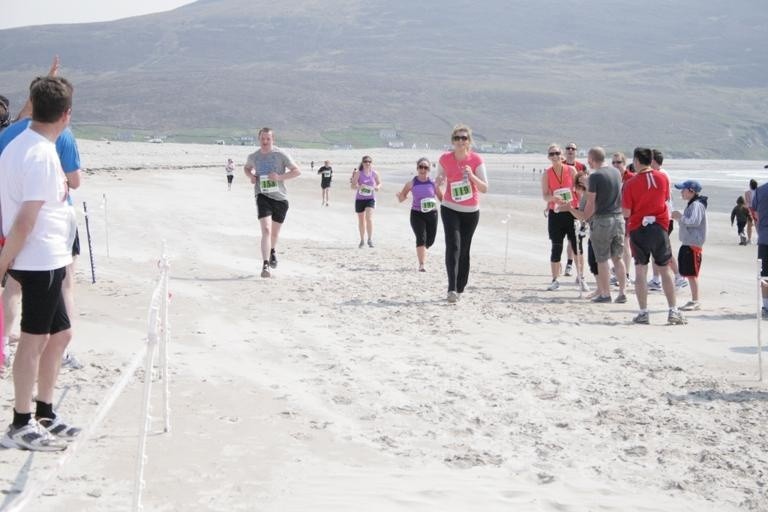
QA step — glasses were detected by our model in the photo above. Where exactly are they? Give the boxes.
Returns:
[566,147,576,151]
[548,152,561,156]
[455,136,467,141]
[419,165,428,169]
[612,160,622,164]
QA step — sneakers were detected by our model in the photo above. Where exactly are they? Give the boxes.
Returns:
[269,248,277,268]
[548,279,559,290]
[633,312,649,323]
[668,309,688,324]
[680,301,700,311]
[261,260,270,277]
[576,277,590,292]
[0,416,67,452]
[34,410,82,441]
[614,294,626,303]
[447,291,457,302]
[591,294,612,303]
[565,263,573,276]
[647,280,662,290]
[675,280,688,288]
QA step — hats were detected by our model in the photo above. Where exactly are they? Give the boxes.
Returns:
[674,180,702,192]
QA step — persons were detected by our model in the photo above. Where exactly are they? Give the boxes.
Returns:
[730,164,768,320]
[0,53,82,453]
[350,156,380,248]
[395,158,443,272]
[318,161,333,206]
[434,124,488,302]
[244,128,301,278]
[311,161,314,169]
[542,142,707,325]
[225,159,234,190]
